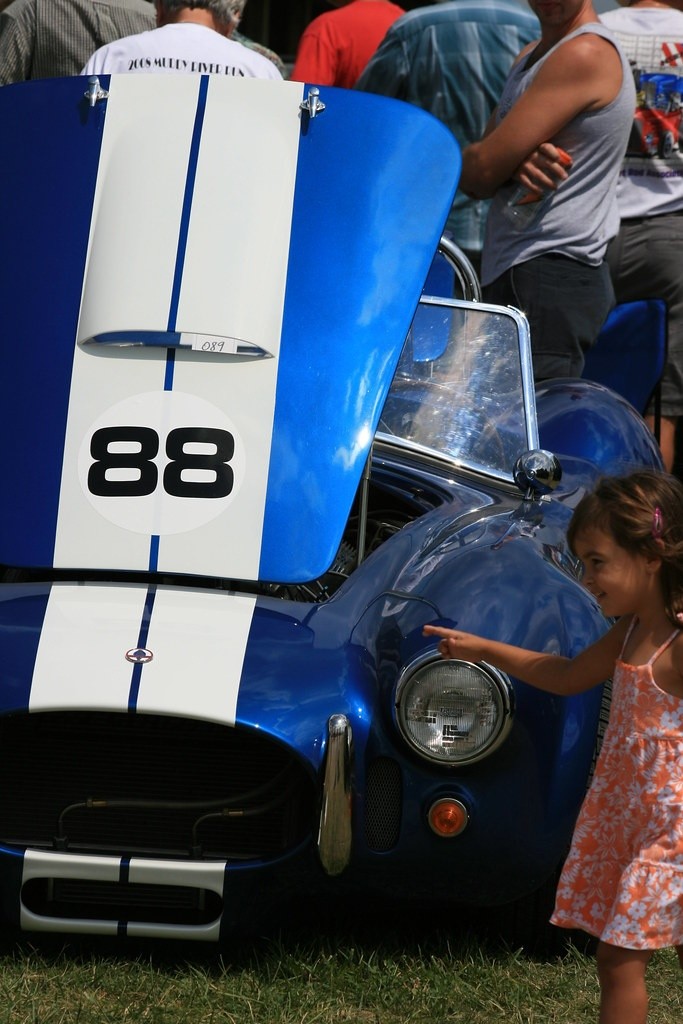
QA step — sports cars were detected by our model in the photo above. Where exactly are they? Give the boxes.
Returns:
[0,74,681,960]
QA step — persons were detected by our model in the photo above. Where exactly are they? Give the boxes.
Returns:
[356,0,542,326]
[0,0,288,86]
[460,0,636,383]
[598,0,683,473]
[290,0,406,90]
[422,469,683,1024]
